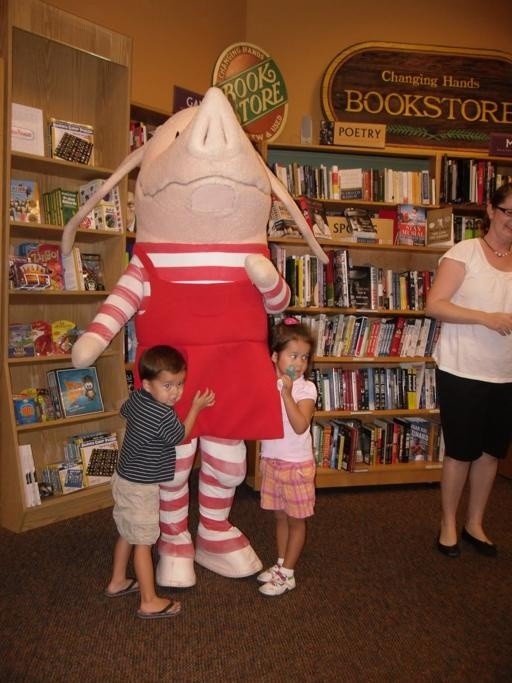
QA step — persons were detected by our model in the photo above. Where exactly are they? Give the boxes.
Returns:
[256,321,319,597]
[102,343,218,617]
[420,181,510,557]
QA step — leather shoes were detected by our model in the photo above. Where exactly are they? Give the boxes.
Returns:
[461,525,497,556]
[437,527,460,558]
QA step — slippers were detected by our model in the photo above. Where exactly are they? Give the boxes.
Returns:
[136,597,182,619]
[103,577,140,598]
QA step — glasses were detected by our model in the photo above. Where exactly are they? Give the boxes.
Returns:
[494,205,512,216]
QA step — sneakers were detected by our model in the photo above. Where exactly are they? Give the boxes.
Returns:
[256,562,281,582]
[258,570,297,596]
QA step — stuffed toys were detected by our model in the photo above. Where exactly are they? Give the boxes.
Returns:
[59,84,333,590]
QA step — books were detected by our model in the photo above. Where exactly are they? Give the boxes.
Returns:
[12,366,103,425]
[268,157,511,248]
[11,320,82,358]
[479,234,511,257]
[19,432,121,505]
[270,243,446,472]
[10,242,106,292]
[10,105,95,166]
[11,179,121,230]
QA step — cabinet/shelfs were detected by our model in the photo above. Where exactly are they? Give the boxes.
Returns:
[119,101,177,375]
[241,137,511,496]
[0,0,133,535]
